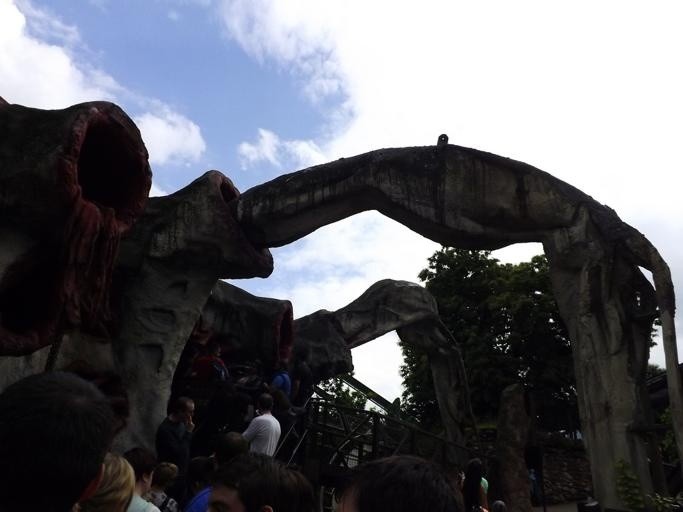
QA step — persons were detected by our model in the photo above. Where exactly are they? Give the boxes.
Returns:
[335,457,507,510]
[2,339,315,511]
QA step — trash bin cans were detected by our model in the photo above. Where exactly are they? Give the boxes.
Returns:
[577,502,601,512]
